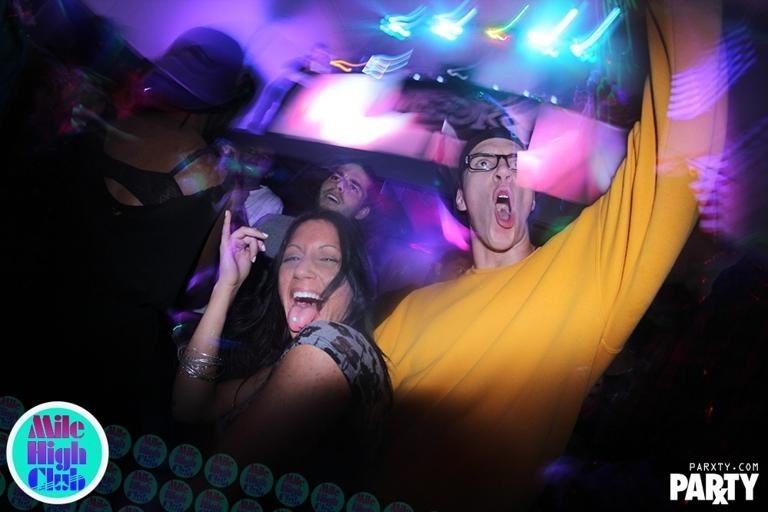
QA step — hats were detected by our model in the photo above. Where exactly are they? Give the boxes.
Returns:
[460,127,526,172]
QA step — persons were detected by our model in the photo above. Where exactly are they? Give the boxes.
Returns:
[79,0,730,512]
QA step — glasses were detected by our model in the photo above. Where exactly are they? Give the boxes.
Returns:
[462,153,519,174]
